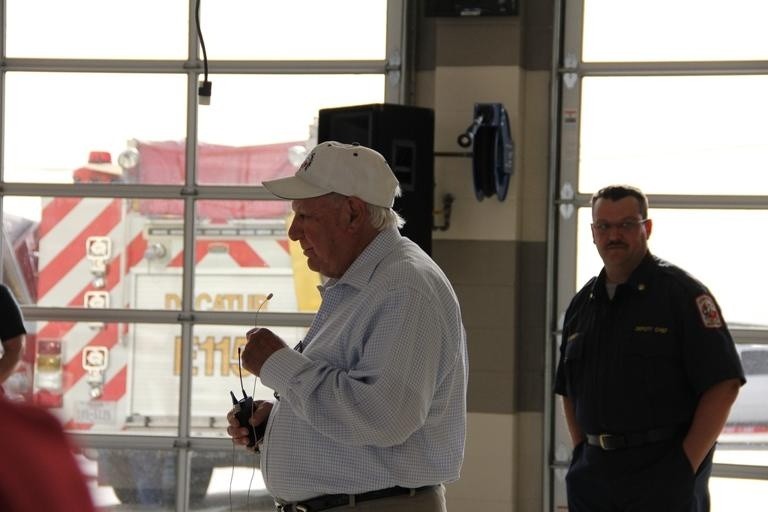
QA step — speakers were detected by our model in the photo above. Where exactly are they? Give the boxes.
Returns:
[316,103,435,255]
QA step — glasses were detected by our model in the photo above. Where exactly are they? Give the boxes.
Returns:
[596,219,649,230]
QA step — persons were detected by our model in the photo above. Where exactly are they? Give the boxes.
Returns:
[224,139,473,511]
[1,282,28,384]
[555,182,748,512]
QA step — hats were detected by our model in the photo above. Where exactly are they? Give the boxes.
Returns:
[262,140,399,209]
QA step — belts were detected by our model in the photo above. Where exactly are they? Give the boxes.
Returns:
[585,433,652,449]
[273,485,430,511]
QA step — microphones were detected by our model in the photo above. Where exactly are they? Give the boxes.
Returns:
[255,293,273,329]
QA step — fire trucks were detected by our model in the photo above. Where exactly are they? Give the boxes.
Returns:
[32,117,329,505]
